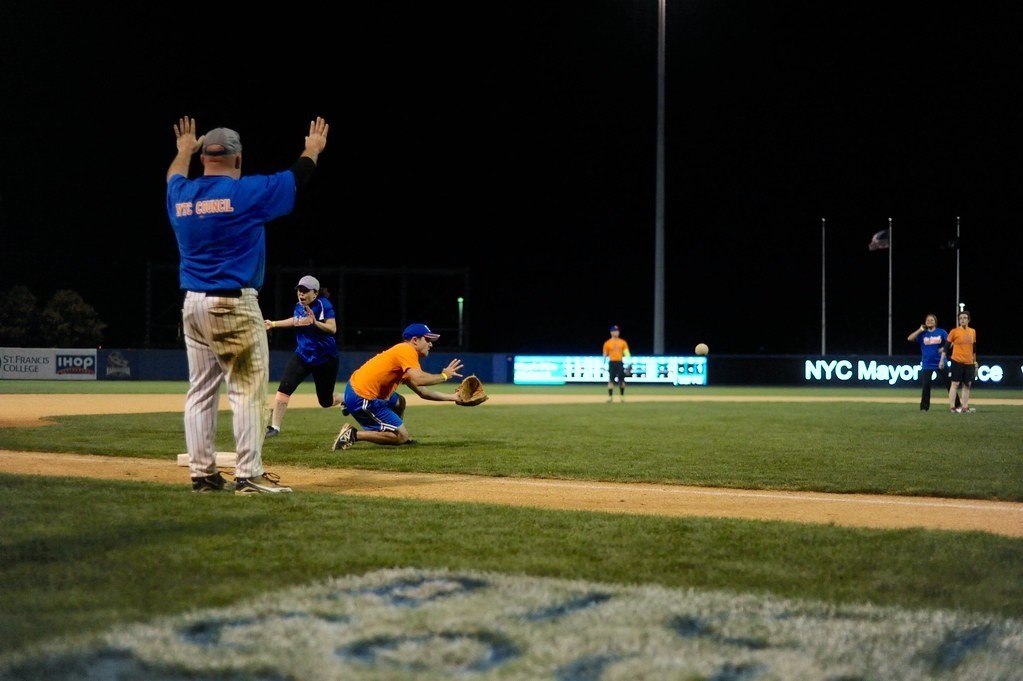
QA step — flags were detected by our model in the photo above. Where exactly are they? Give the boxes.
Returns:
[868,229,890,249]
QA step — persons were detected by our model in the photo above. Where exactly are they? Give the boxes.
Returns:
[265,275,344,438]
[603,325,630,403]
[166,117,328,496]
[907,312,978,412]
[332,324,463,451]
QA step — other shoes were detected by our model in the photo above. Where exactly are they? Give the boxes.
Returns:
[606,399,626,403]
[949,408,976,413]
[920,410,928,413]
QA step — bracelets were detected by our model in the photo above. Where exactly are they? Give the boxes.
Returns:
[271,321,275,328]
[974,362,977,364]
[441,373,448,382]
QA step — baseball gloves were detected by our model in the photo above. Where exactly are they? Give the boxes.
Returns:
[624,366,632,376]
[455,375,489,406]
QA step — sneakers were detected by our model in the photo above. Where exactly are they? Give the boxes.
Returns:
[332,422,356,451]
[191,472,236,492]
[234,473,292,497]
[406,440,416,444]
[340,402,350,416]
[265,426,280,438]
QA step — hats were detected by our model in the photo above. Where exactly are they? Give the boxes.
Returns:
[295,275,320,291]
[610,325,619,331]
[202,127,243,155]
[403,323,441,341]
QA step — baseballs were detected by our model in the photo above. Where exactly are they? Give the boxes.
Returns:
[695,343,708,355]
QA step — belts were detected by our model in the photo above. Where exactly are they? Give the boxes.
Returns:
[206,289,242,299]
